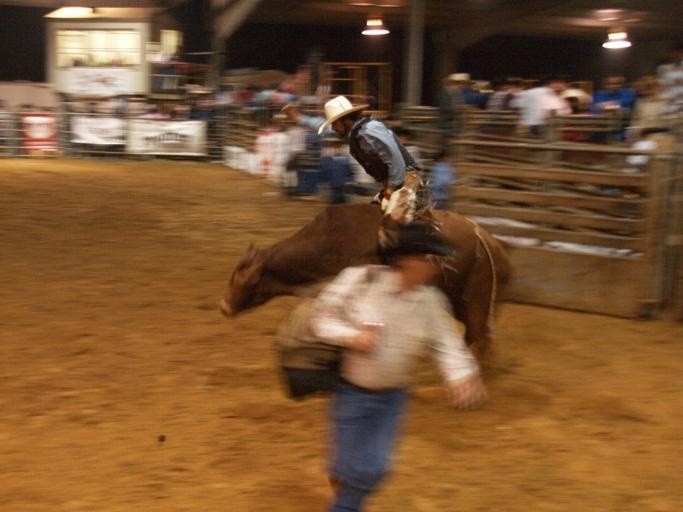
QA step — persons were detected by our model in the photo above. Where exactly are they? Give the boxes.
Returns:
[310,223,488,511]
[317,95,420,243]
[444,48,683,172]
[430,149,453,209]
[283,104,350,205]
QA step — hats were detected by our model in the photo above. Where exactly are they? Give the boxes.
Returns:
[317,95,370,137]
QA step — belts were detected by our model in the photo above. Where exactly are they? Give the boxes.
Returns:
[336,375,407,399]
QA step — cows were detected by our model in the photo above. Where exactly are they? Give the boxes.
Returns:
[219,203,514,359]
